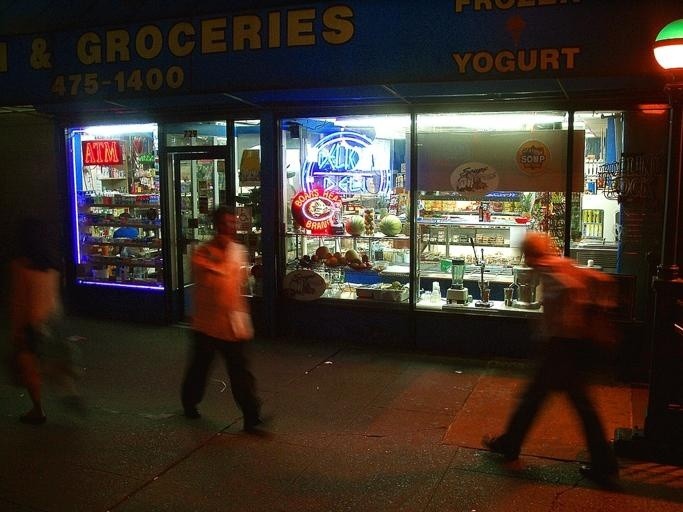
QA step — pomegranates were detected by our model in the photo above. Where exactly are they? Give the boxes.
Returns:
[316,246,327,258]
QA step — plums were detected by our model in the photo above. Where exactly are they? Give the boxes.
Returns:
[364,209,374,234]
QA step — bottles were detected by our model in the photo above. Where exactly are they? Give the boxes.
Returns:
[77,191,162,288]
[585,172,614,195]
[131,148,159,192]
[478,202,491,221]
[422,281,442,304]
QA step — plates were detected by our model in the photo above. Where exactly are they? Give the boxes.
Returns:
[448,162,500,198]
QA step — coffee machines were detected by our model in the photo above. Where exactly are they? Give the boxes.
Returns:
[510,265,542,311]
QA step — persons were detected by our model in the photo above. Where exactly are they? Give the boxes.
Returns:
[479,229,622,487]
[2,218,89,426]
[111,212,138,241]
[180,204,276,439]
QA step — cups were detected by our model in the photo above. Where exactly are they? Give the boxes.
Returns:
[503,288,513,306]
[587,260,593,267]
[481,289,490,302]
[315,272,331,283]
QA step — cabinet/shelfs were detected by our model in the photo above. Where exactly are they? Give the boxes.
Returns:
[283,231,412,291]
[415,218,528,300]
[78,202,164,287]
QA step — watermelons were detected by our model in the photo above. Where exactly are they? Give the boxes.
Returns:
[346,215,365,235]
[379,215,402,236]
[345,249,357,261]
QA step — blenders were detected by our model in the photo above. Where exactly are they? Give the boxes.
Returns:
[445,258,471,305]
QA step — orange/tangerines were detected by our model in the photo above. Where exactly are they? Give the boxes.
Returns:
[328,256,338,264]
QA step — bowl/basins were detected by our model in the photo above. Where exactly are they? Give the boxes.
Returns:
[515,218,529,224]
